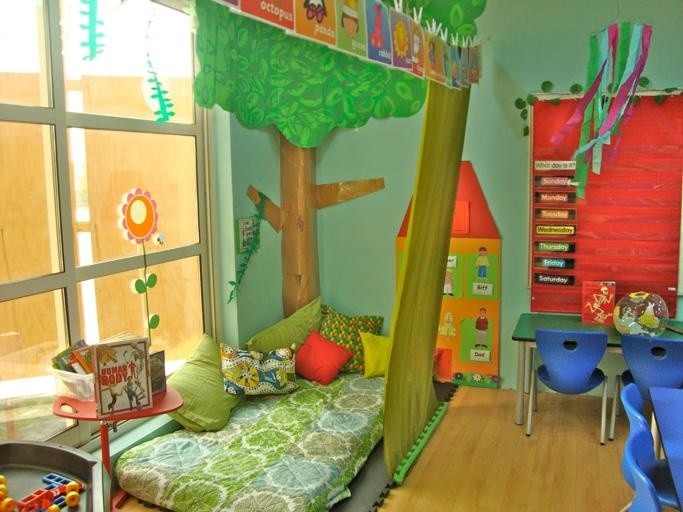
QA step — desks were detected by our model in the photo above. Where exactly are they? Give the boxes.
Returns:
[54,386,183,512]
[649,386,683,512]
[512,314,683,425]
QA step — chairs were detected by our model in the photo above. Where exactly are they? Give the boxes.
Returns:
[624,427,664,512]
[617,383,680,512]
[610,333,683,441]
[526,328,608,445]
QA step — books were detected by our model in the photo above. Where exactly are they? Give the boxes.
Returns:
[51,329,167,421]
[580,279,617,326]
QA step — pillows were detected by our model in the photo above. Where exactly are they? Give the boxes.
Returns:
[320,305,384,374]
[244,295,322,356]
[359,331,391,378]
[295,330,353,386]
[165,331,245,433]
[220,342,300,396]
